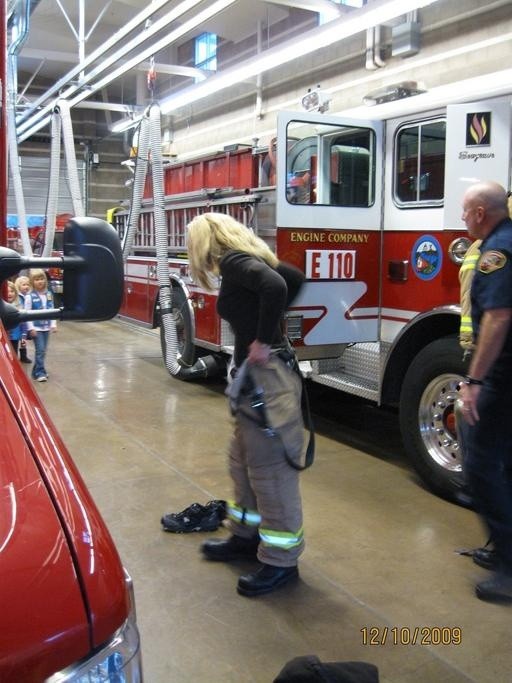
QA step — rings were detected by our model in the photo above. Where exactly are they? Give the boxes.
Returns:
[464,407,470,412]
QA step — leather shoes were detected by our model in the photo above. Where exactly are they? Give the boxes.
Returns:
[473,547,497,571]
[201,535,257,560]
[476,575,512,602]
[236,564,298,596]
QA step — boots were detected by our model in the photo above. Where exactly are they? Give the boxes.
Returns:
[20,348,31,363]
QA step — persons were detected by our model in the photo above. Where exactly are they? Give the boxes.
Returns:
[187,212,303,597]
[452,180,510,605]
[15,275,32,363]
[24,268,59,382]
[8,280,28,356]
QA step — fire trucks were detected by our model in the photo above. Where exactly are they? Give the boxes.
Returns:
[0,0,146,681]
[9,212,77,293]
[107,63,512,506]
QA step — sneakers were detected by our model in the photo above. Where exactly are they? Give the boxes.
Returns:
[161,503,219,533]
[33,377,47,382]
[205,499,226,522]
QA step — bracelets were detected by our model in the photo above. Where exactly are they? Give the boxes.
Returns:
[253,338,272,350]
[463,375,484,385]
